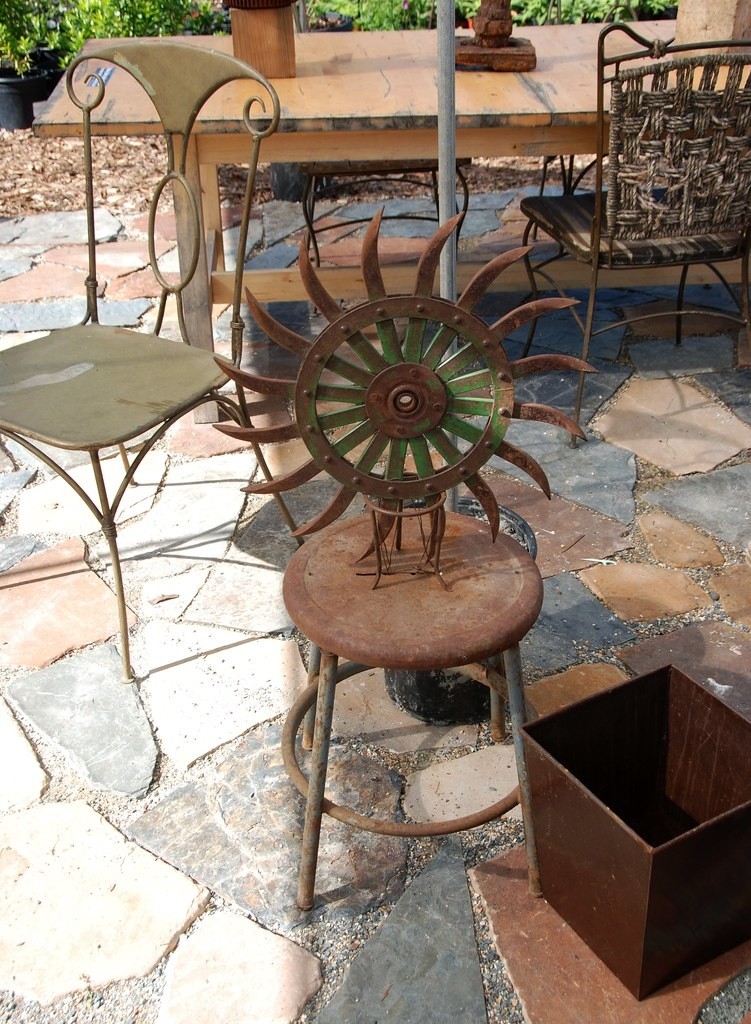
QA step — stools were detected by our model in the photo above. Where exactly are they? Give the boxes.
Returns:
[281,508,544,908]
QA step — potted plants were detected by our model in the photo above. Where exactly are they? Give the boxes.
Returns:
[0,0,515,131]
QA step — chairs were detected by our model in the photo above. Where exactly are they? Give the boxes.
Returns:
[289,1,751,448]
[0,43,303,685]
[523,23,751,446]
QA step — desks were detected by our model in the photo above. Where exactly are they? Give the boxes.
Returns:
[34,21,751,426]
